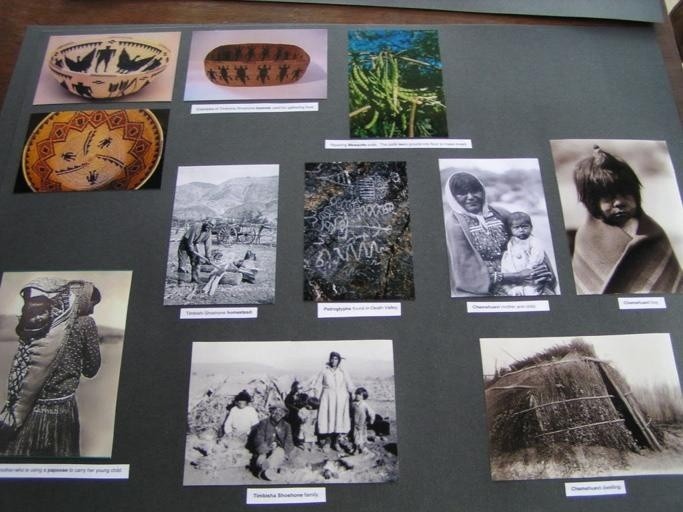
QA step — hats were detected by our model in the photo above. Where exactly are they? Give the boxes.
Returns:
[234,390,251,402]
[267,402,289,415]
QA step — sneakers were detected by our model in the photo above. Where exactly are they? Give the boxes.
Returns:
[321,443,330,454]
[332,443,344,453]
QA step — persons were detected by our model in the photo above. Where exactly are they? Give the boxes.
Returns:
[444,171,558,296]
[284,380,310,446]
[177,218,217,287]
[254,402,293,482]
[223,389,262,435]
[571,144,683,296]
[316,351,357,452]
[500,211,545,296]
[12,280,102,456]
[297,398,320,453]
[22,296,50,329]
[350,388,376,453]
